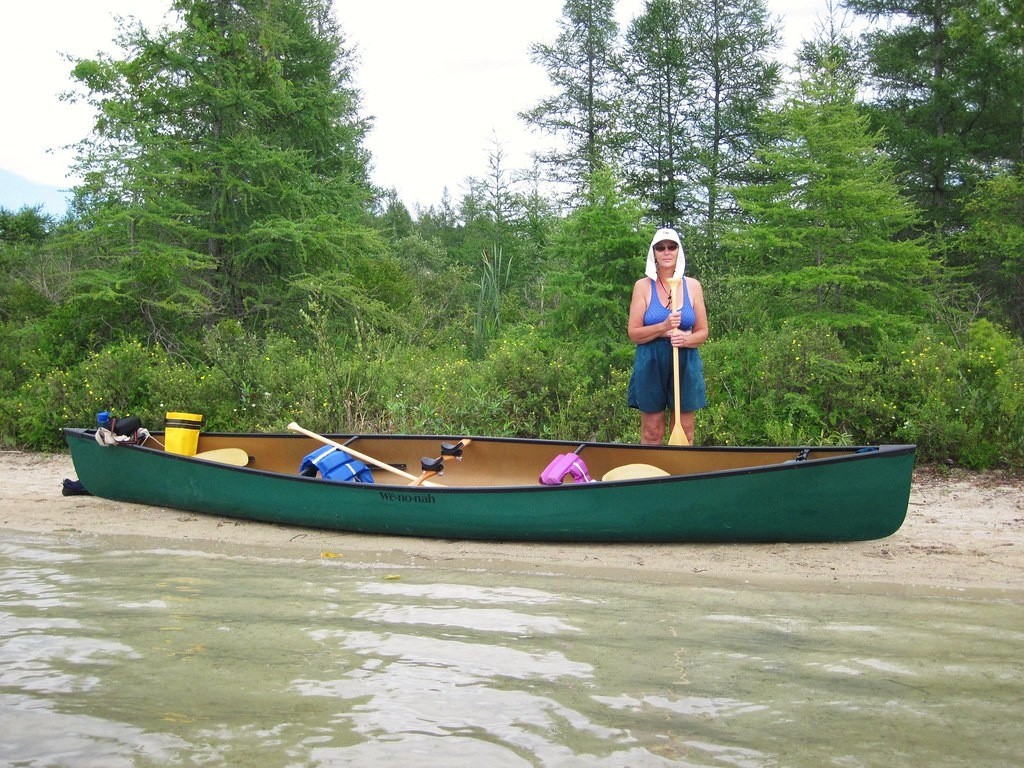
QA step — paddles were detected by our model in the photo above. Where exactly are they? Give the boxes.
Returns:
[667,277,690,447]
[286,422,448,488]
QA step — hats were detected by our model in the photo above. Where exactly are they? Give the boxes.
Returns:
[645,228,685,281]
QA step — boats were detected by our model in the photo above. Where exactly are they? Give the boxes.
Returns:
[63,434,917,545]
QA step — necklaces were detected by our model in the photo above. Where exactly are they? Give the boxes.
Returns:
[656,271,676,309]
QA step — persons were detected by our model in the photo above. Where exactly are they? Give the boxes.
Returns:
[628,229,708,446]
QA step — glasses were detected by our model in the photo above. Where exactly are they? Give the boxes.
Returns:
[654,244,677,251]
